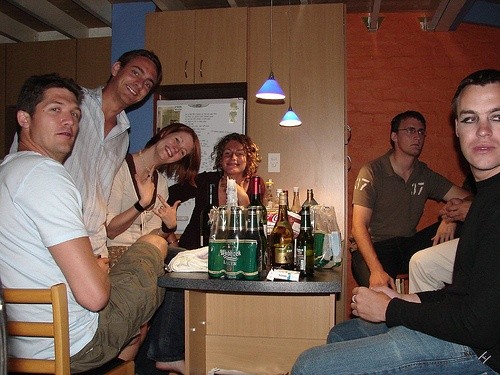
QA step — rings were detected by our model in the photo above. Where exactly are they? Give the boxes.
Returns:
[148,175,152,178]
[352,295,356,303]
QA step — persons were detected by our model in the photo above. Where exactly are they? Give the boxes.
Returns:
[349,111,473,288]
[166,133,266,248]
[290,69,500,375]
[0,73,169,374]
[106,123,201,375]
[409,195,473,294]
[9,49,163,259]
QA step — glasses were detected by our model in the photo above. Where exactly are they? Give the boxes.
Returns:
[399,128,427,137]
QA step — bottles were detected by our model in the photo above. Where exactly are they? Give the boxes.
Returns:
[250,175,268,237]
[302,187,319,209]
[290,186,301,214]
[207,204,268,279]
[268,190,296,269]
[294,206,316,278]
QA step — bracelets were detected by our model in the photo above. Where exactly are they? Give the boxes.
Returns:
[169,240,178,244]
[162,224,177,233]
[134,202,144,212]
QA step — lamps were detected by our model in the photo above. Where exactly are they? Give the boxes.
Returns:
[279,34,302,127]
[256,0,286,100]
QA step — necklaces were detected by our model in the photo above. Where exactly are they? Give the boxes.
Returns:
[139,151,147,169]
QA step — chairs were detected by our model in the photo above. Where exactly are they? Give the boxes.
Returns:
[0,283,135,375]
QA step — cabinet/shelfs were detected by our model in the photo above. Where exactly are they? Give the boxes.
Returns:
[146,4,353,375]
[5,36,111,107]
